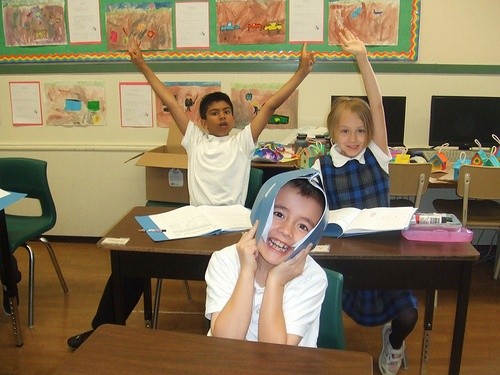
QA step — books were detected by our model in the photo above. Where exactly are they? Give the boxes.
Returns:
[134,204,254,243]
[324,206,419,239]
[250,168,330,264]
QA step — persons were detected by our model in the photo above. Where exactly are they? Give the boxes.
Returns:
[204,168,329,349]
[0,209,22,316]
[67,36,315,349]
[309,27,420,375]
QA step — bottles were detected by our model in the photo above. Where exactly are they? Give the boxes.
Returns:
[294,135,307,154]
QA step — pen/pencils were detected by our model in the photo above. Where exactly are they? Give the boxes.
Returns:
[139,229,166,232]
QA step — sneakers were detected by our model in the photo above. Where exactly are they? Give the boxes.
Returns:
[379,322,406,375]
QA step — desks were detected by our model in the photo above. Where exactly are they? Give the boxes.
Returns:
[60,324,373,375]
[97,206,480,375]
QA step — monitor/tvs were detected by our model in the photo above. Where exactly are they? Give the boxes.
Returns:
[331,96,406,147]
[429,96,500,152]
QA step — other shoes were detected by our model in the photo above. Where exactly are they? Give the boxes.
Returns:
[2,283,19,315]
[67,330,93,348]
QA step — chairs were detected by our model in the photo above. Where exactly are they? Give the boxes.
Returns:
[433,165,500,279]
[317,266,346,349]
[387,162,432,212]
[0,157,68,330]
[153,167,265,330]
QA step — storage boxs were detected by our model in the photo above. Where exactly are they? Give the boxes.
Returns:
[124,119,190,204]
